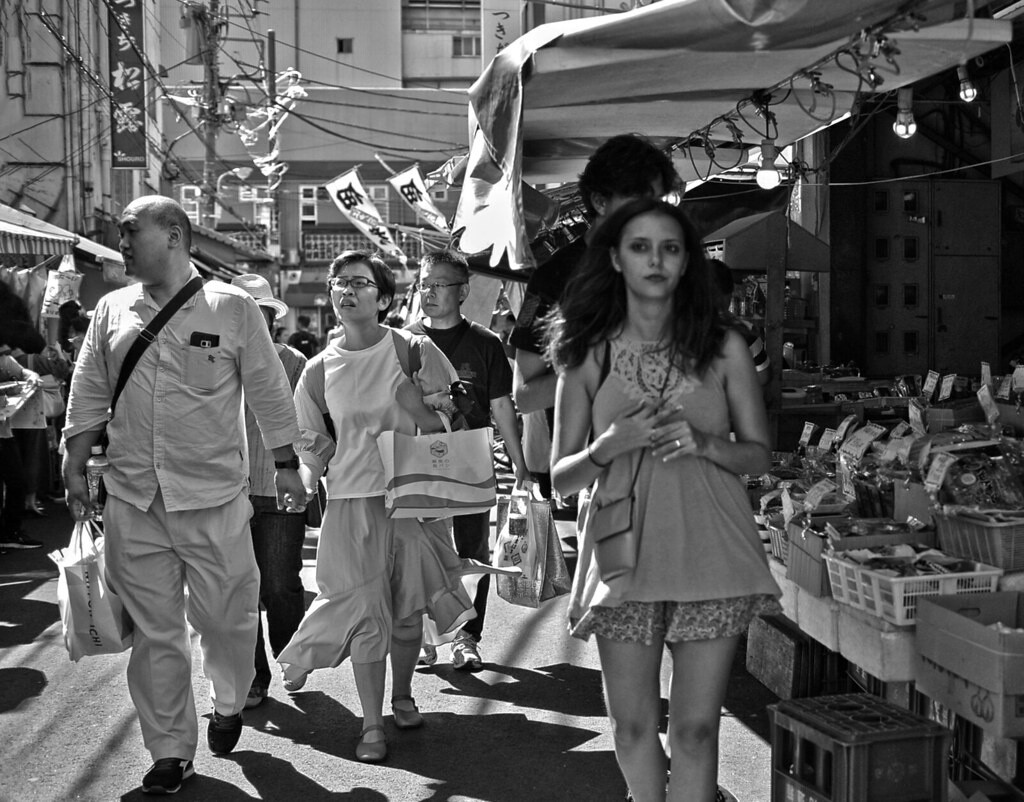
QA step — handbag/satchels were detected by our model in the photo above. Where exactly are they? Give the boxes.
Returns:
[594,496,637,582]
[377,410,497,519]
[306,478,327,528]
[48,513,133,663]
[27,353,65,418]
[493,479,572,609]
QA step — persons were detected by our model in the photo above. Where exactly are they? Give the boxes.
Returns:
[0,286,106,550]
[229,274,416,707]
[548,198,782,802]
[285,249,466,762]
[403,249,534,670]
[58,195,308,792]
[509,134,770,718]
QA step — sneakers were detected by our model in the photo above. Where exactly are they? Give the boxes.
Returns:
[141,758,194,794]
[448,630,482,670]
[245,686,268,709]
[0,530,44,549]
[283,668,313,690]
[416,644,438,665]
[207,707,242,755]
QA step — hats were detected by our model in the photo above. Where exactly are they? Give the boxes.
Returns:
[230,274,289,319]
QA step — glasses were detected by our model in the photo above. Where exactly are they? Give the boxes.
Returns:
[328,275,386,292]
[415,282,466,292]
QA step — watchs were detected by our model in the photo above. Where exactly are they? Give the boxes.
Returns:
[275,456,300,470]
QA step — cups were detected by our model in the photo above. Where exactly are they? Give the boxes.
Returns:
[509,490,528,535]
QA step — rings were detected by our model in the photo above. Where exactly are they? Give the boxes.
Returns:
[305,500,308,505]
[676,440,681,448]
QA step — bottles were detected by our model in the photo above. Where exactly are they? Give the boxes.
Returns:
[806,385,824,404]
[730,280,808,320]
[86,446,110,521]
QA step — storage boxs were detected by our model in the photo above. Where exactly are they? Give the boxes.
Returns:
[767,362,1024,802]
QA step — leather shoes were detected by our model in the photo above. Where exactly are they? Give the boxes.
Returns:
[355,725,387,763]
[391,695,424,727]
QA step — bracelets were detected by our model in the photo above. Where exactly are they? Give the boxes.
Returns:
[589,452,613,467]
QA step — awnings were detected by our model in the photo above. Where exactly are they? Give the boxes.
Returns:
[451,0,976,272]
[0,203,132,286]
